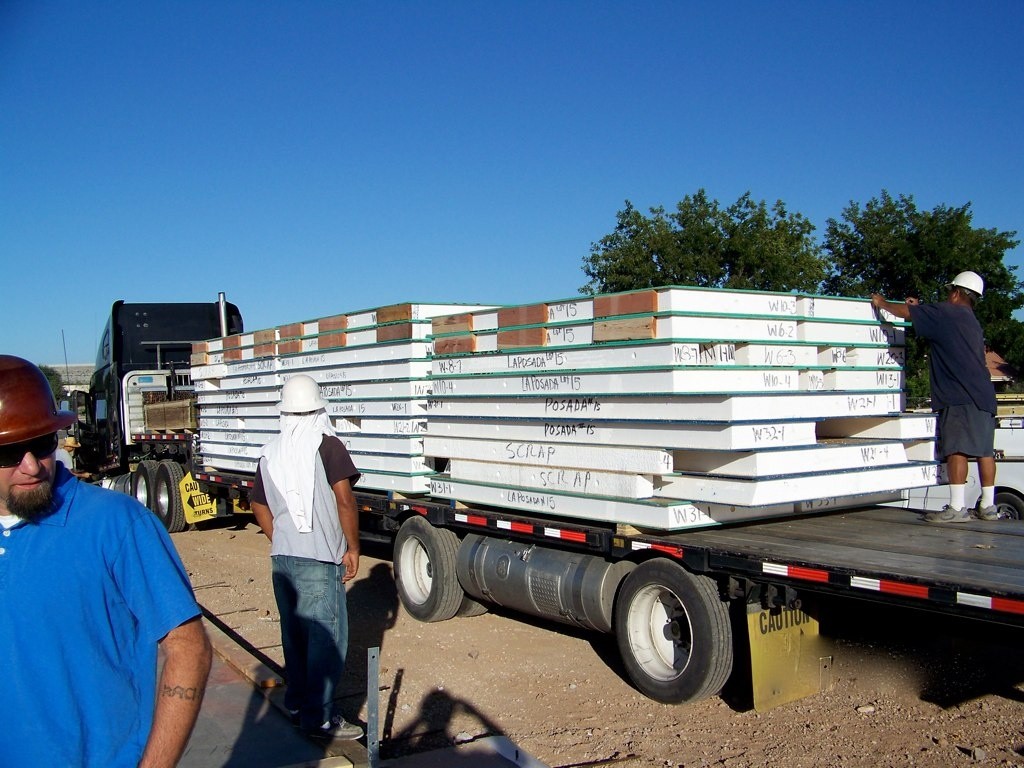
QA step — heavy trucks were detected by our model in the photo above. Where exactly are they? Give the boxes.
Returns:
[79,291,1024,706]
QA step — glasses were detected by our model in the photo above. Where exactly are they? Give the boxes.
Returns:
[1,432,58,470]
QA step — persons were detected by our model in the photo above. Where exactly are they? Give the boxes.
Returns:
[250,376,363,739]
[0,355,210,768]
[872,271,997,522]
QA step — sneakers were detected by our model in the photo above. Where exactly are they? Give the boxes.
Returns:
[288,708,309,728]
[302,713,364,741]
[977,504,997,520]
[923,507,970,522]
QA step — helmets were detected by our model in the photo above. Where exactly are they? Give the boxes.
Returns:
[944,271,984,296]
[1,355,76,447]
[275,375,329,413]
